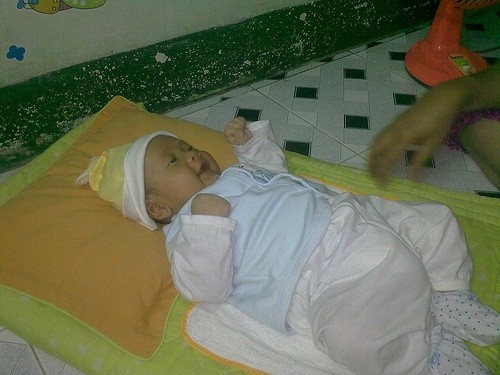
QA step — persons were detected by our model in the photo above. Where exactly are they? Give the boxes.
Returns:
[367,61,500,193]
[76,115,500,375]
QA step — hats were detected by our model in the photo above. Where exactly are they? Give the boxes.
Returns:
[73,131,184,231]
